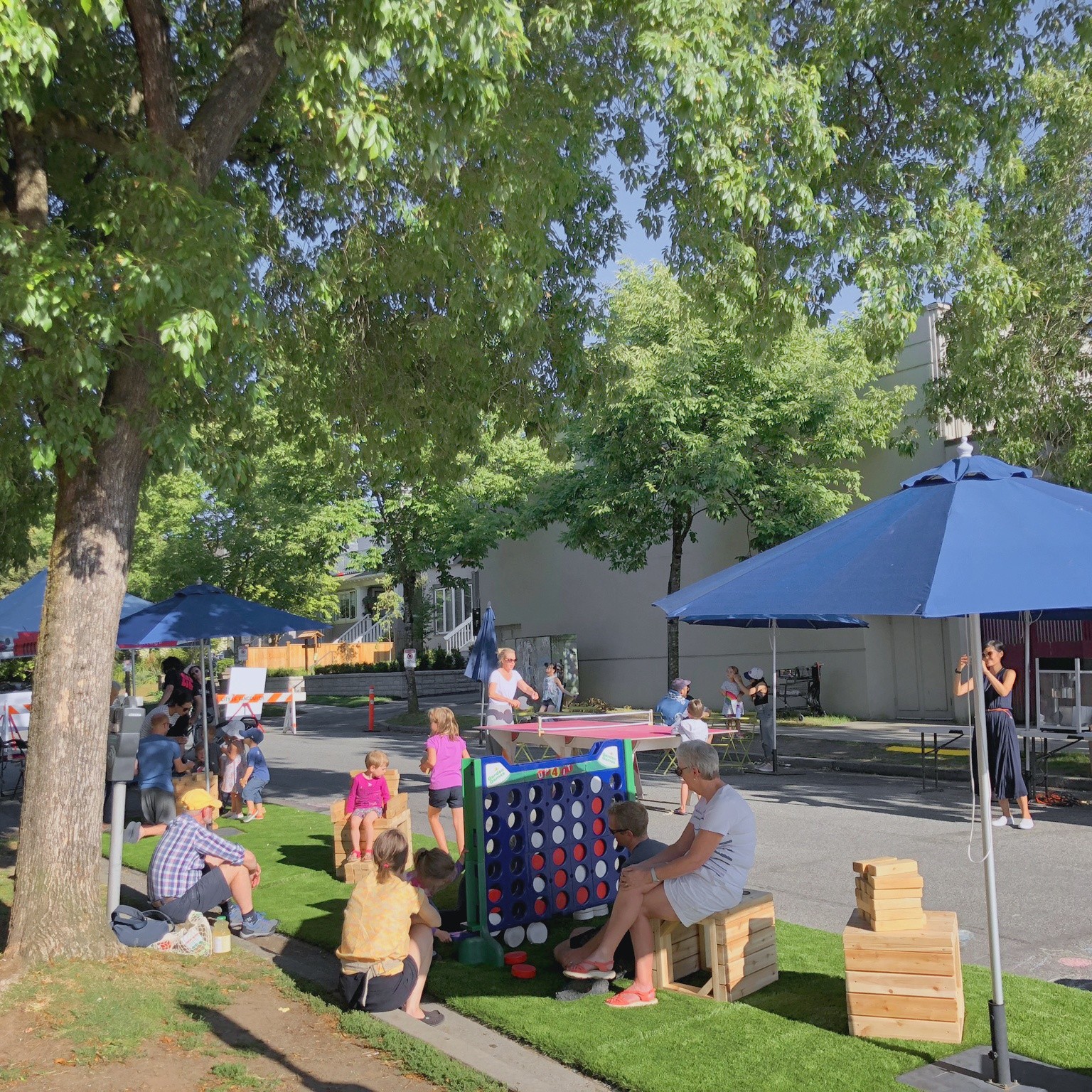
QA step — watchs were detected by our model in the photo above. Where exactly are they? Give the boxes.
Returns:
[955,668,962,674]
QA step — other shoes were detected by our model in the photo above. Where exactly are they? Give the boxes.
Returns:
[417,1008,445,1027]
[124,821,143,845]
[755,761,773,772]
[1018,818,1034,829]
[221,811,244,819]
[992,816,1014,827]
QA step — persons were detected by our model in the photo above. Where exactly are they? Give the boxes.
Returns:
[654,678,713,746]
[718,666,745,738]
[538,662,576,722]
[335,829,445,1028]
[486,648,539,766]
[147,789,282,941]
[552,800,670,979]
[953,640,1034,829]
[123,656,270,846]
[732,667,774,772]
[419,707,471,858]
[401,846,466,961]
[343,750,391,863]
[669,699,709,815]
[563,739,756,1009]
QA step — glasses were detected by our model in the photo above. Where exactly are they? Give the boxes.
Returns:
[180,706,192,712]
[505,658,518,663]
[608,826,631,835]
[982,651,995,658]
[162,722,173,728]
[191,670,200,676]
[674,766,690,776]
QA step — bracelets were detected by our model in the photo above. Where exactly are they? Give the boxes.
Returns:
[650,866,660,882]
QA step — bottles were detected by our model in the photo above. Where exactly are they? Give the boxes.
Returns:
[7,742,13,760]
[736,705,741,718]
[212,916,231,953]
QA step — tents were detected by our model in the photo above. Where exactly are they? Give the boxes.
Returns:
[0,569,218,753]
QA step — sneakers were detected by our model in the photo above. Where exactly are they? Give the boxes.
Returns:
[220,903,267,928]
[239,911,280,940]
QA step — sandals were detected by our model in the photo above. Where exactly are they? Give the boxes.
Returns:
[562,959,616,980]
[603,988,658,1008]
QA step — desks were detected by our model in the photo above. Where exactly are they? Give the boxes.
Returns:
[472,714,739,800]
[700,712,755,736]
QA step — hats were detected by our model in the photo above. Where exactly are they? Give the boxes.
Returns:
[743,667,763,680]
[180,789,222,810]
[221,720,245,740]
[239,728,263,744]
[544,662,557,673]
[671,678,691,692]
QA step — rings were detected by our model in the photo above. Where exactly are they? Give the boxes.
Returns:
[570,962,572,965]
[625,883,628,887]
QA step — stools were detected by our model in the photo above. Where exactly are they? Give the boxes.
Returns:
[636,884,783,1008]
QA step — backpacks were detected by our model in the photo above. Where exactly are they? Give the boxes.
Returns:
[108,905,175,948]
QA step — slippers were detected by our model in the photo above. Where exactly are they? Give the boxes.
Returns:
[243,808,264,823]
[673,807,688,816]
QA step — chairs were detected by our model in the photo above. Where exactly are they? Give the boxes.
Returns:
[328,767,421,879]
[659,709,762,779]
[0,735,30,800]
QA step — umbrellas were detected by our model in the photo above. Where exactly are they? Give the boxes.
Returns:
[678,614,870,775]
[464,600,503,748]
[115,577,333,795]
[650,439,1092,1092]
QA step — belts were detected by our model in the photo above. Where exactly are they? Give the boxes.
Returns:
[149,897,177,908]
